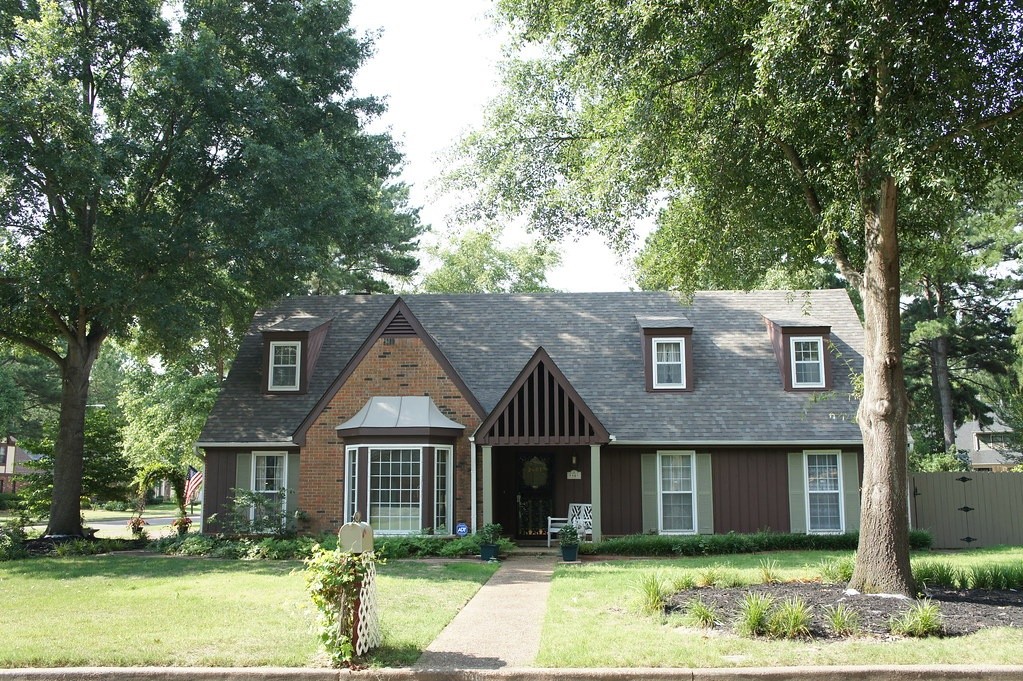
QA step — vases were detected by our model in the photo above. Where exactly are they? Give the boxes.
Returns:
[177,526,188,534]
[132,527,143,534]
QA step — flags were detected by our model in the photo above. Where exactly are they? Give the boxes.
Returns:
[182,465,202,506]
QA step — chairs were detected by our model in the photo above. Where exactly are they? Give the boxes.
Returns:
[547,503,593,548]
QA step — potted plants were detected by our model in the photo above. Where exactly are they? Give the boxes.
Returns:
[558,524,580,562]
[479,523,503,560]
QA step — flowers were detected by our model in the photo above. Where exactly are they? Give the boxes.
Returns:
[173,516,193,526]
[128,518,146,527]
